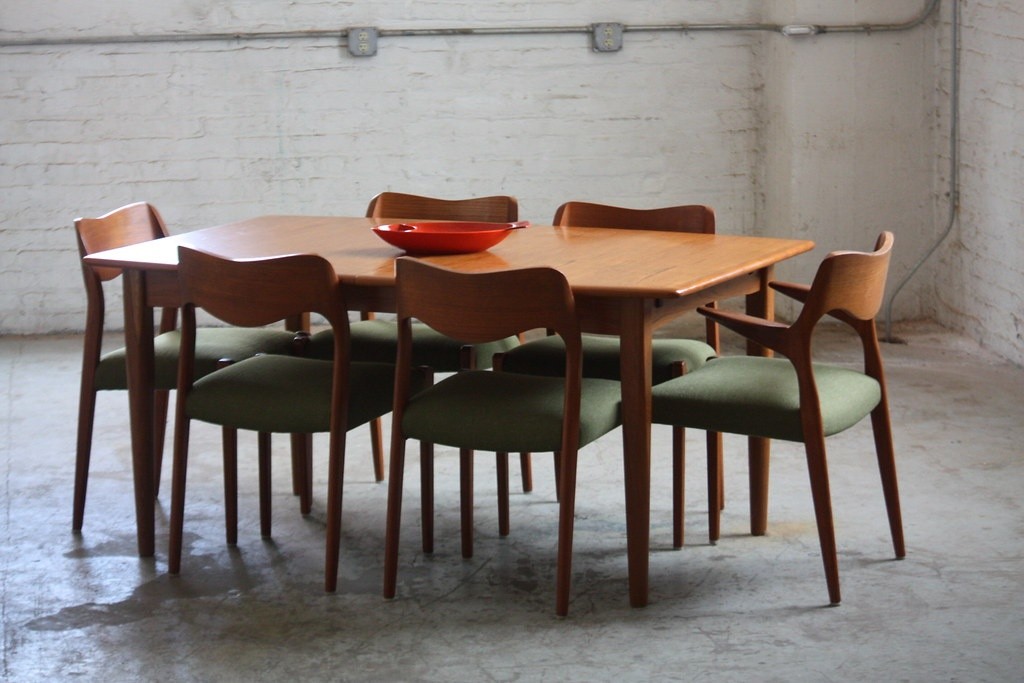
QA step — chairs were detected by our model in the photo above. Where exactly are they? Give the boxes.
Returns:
[73,192,534,599]
[384,256,623,620]
[651,231,906,605]
[493,202,726,548]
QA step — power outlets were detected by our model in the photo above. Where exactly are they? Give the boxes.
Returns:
[591,22,623,52]
[347,27,378,57]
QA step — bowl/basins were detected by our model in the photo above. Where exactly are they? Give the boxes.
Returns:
[371,221,531,254]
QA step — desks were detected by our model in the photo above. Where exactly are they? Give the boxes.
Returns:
[83,215,817,609]
